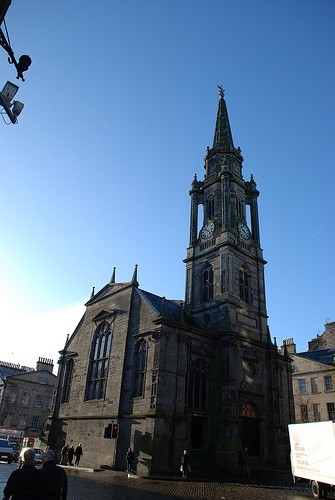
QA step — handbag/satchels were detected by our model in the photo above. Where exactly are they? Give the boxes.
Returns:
[188,465,192,472]
[181,465,184,471]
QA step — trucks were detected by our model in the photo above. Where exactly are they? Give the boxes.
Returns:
[287,420,335,500]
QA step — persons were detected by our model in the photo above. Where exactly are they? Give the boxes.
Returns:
[38,450,68,500]
[242,447,251,477]
[125,447,135,473]
[59,444,69,465]
[67,445,75,466]
[1,447,47,500]
[74,443,83,467]
[180,450,191,479]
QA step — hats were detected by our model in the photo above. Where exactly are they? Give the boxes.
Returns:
[43,449,55,460]
[23,449,36,464]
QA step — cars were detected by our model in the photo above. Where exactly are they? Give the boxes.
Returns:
[0,438,20,464]
[32,447,45,466]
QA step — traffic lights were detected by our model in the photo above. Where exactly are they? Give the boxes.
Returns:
[112,424,118,438]
